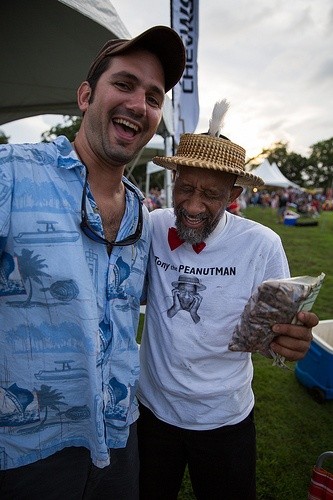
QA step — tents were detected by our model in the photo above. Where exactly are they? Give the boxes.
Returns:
[243,159,304,194]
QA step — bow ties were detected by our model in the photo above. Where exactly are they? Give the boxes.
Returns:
[168,227,206,254]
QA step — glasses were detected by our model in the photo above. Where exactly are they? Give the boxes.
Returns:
[78,180,143,247]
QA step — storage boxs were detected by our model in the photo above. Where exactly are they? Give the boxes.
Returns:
[283,215,296,225]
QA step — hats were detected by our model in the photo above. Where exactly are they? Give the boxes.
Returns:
[86,25,186,95]
[152,97,265,187]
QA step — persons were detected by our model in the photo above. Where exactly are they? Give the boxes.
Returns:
[144,180,165,211]
[136,99,319,500]
[0,25,189,500]
[229,184,333,229]
